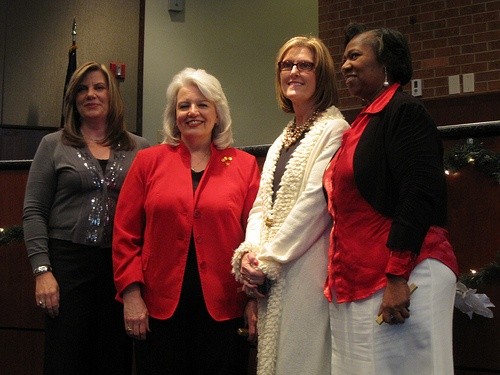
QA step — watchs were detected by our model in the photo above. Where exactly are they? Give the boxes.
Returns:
[32,265,53,274]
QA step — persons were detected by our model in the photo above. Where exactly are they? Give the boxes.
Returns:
[322,20,459,375]
[232,36,350,375]
[111,66,262,375]
[22,61,152,375]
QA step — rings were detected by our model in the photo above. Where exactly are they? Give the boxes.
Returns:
[128,327,133,331]
[39,301,46,305]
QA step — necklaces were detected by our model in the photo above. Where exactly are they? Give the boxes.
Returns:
[283,109,320,150]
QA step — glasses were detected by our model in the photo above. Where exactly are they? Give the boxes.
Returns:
[278,61,314,72]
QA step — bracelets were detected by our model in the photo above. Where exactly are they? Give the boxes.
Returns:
[33,271,47,277]
